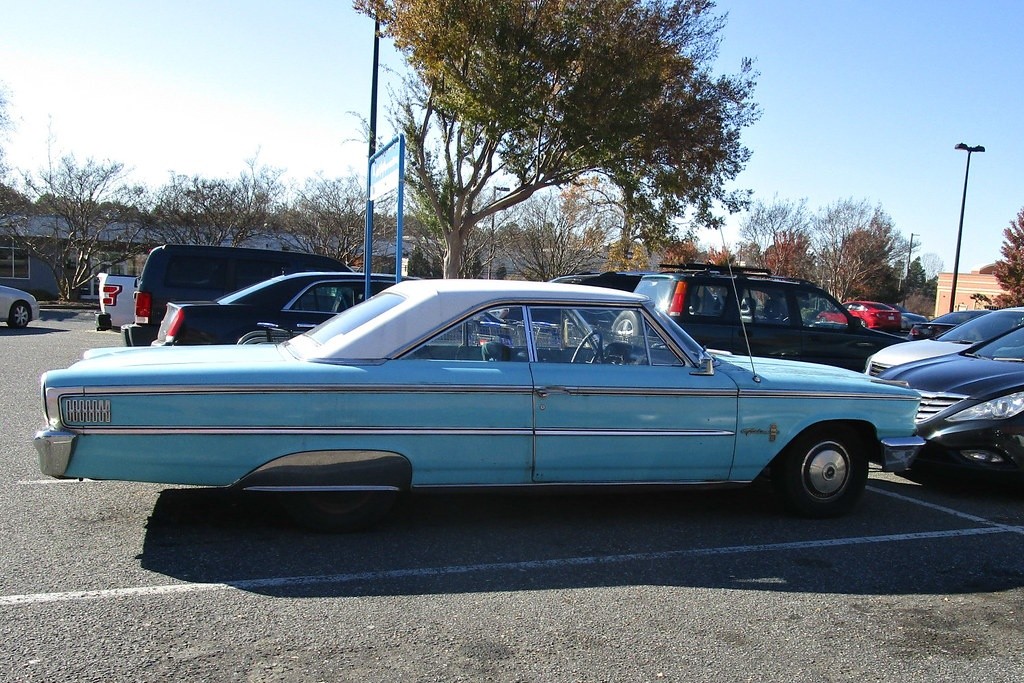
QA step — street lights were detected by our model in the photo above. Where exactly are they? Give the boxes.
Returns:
[950,143,984,312]
[487,186,511,277]
[903,234,921,306]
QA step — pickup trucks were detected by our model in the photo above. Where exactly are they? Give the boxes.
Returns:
[96,273,139,332]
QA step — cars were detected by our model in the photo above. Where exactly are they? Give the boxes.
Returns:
[149,271,566,347]
[863,305,1024,376]
[878,320,1024,487]
[31,276,927,517]
[814,301,902,332]
[889,303,927,331]
[908,310,992,340]
[477,272,643,341]
[0,286,39,328]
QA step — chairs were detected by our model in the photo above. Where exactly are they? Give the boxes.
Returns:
[741,298,757,322]
[482,343,511,363]
[762,299,783,323]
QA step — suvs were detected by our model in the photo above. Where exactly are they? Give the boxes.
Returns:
[123,244,368,348]
[613,271,911,372]
